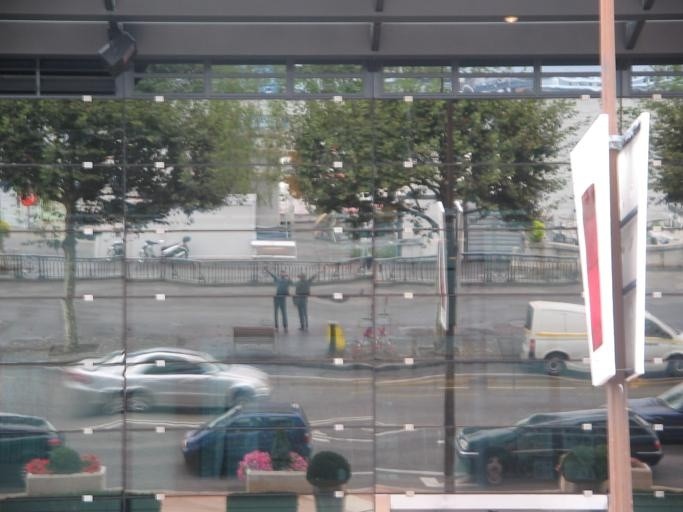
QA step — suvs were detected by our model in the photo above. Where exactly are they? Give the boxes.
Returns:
[455,405,664,487]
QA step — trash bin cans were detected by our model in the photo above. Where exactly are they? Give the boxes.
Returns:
[328,322,346,351]
[281,221,291,238]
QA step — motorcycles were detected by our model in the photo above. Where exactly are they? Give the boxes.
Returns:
[137,235,191,264]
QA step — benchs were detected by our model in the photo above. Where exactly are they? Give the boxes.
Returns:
[235,327,278,353]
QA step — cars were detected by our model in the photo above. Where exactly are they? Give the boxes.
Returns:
[178,401,311,472]
[58,347,272,416]
[552,233,576,246]
[0,411,61,489]
[627,381,682,443]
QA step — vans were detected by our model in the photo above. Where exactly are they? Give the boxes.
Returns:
[518,299,682,376]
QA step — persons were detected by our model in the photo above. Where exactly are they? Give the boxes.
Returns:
[273,429,293,470]
[288,271,317,330]
[265,267,291,333]
